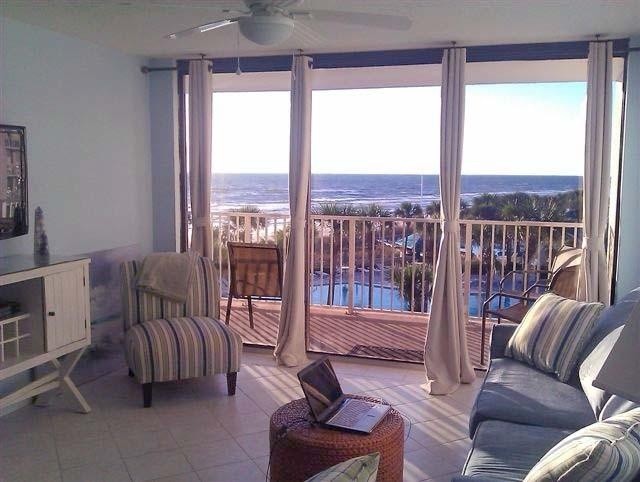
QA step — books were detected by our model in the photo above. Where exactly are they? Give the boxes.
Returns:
[0,302,22,318]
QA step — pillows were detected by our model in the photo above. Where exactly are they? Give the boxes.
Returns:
[503,293,640,482]
[303,452,380,481]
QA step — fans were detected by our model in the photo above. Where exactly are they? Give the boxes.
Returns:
[161,0,412,76]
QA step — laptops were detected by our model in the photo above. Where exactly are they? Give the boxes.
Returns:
[297,354,392,434]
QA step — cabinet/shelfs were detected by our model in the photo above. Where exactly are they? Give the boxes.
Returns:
[0,253,92,415]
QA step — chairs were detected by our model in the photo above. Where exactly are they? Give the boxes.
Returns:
[481,243,583,364]
[120,242,283,408]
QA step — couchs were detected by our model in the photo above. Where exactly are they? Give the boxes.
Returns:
[450,287,640,482]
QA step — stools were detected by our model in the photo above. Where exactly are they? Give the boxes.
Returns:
[269,393,404,481]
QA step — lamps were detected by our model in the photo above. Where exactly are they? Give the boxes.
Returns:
[236,16,295,48]
